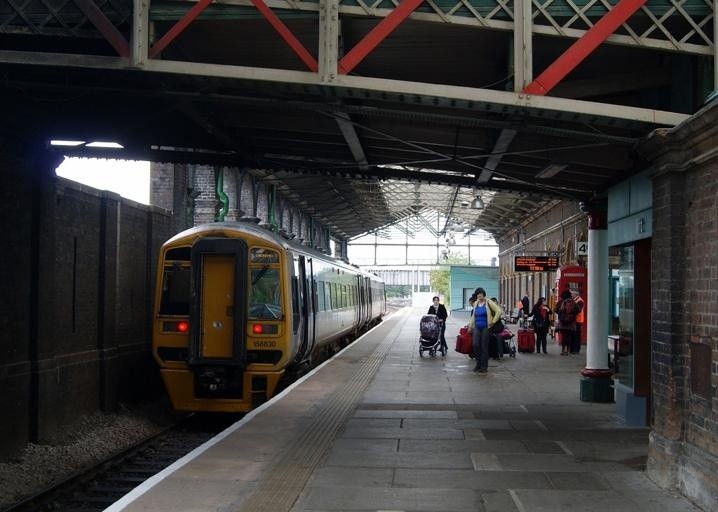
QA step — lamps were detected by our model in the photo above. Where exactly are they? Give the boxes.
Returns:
[470,195,484,209]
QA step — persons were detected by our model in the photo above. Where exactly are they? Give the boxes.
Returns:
[569,287,585,354]
[461,287,506,373]
[520,291,530,322]
[426,296,447,353]
[558,289,580,356]
[552,293,570,347]
[524,297,553,354]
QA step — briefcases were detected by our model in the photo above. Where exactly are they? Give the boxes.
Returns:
[455,325,472,354]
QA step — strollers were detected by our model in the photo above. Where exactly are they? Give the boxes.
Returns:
[499,318,520,361]
[419,314,447,357]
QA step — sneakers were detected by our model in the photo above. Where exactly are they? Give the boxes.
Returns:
[472,368,488,374]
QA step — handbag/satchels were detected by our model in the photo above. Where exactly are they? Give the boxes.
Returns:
[492,311,504,333]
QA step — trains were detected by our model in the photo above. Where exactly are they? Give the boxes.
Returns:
[149,214,390,414]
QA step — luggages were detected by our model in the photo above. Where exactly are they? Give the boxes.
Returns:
[518,318,535,353]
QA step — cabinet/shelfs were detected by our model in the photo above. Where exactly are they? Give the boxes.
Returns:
[607,335,620,375]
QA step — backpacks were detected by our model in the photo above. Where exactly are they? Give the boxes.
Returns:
[559,298,575,323]
